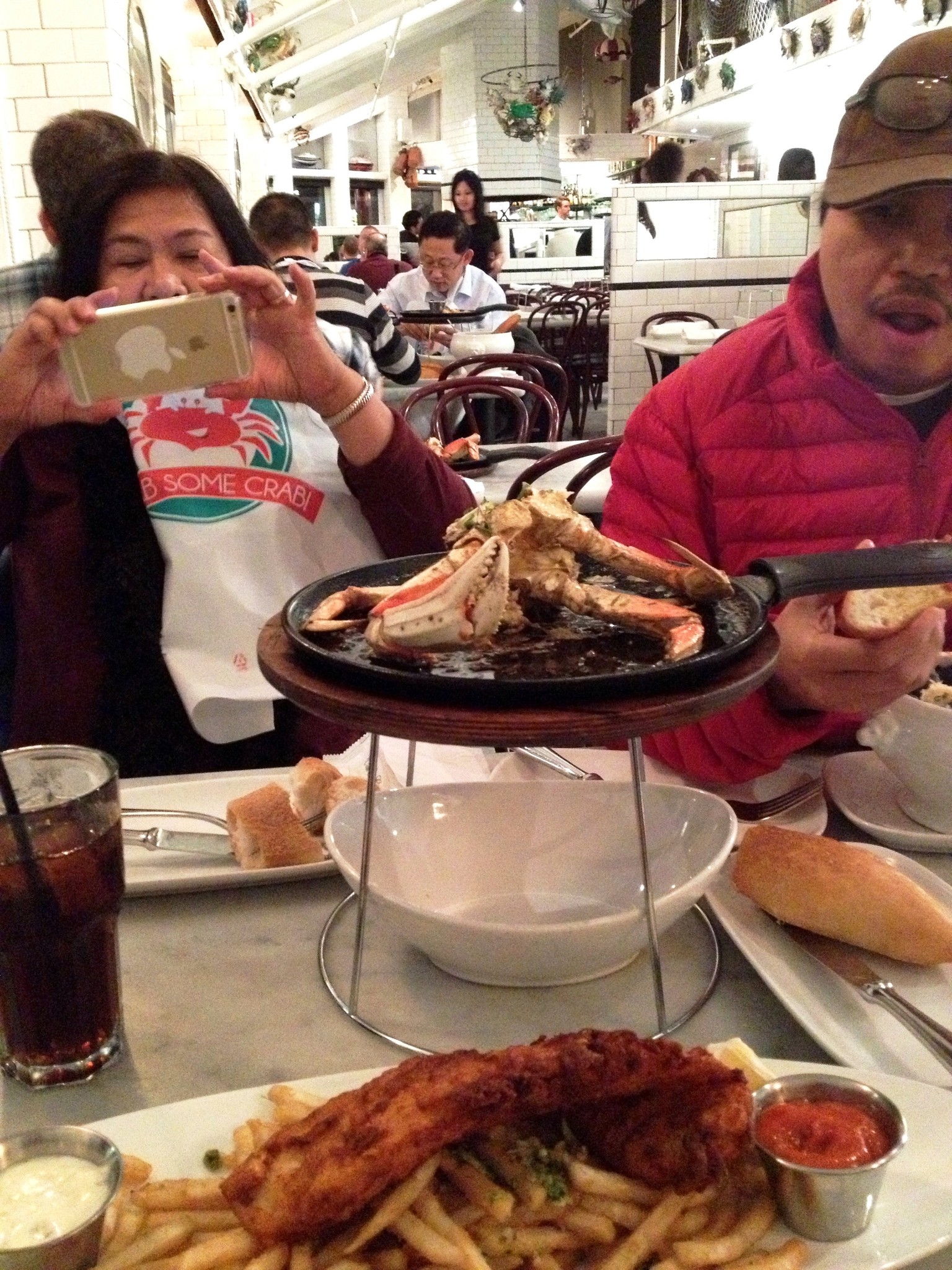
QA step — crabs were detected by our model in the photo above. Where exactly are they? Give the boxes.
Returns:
[296,477,739,665]
[425,432,481,461]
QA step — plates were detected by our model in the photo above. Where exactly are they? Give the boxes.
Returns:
[650,321,710,339]
[113,764,346,898]
[820,749,952,857]
[684,328,730,345]
[686,835,952,1092]
[1,1059,952,1270]
[491,748,830,840]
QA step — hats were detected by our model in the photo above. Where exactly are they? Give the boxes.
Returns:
[821,26,952,209]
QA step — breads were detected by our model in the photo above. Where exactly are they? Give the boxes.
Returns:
[419,363,445,380]
[491,312,522,334]
[227,754,382,870]
[734,821,952,971]
[838,538,950,641]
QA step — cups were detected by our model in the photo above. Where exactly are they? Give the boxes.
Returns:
[3,744,127,1092]
[747,1071,909,1240]
[1,1124,119,1270]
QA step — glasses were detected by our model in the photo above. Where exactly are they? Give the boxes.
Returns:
[846,74,952,131]
[418,250,468,277]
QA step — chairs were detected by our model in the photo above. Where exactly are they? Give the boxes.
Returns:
[505,278,611,441]
[397,352,569,447]
[640,311,719,387]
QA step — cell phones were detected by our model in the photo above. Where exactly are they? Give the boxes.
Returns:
[53,288,253,408]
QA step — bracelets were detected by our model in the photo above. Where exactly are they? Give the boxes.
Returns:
[319,376,373,430]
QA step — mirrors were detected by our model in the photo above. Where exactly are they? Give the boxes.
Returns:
[127,1,158,153]
[726,134,763,182]
[509,227,593,259]
[636,196,812,255]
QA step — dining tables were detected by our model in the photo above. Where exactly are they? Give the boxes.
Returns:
[473,439,613,533]
[378,354,526,444]
[4,745,952,1270]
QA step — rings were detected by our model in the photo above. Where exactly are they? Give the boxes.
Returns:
[270,290,290,307]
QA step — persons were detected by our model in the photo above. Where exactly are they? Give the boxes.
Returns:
[396,208,423,246]
[686,165,720,182]
[452,163,508,277]
[776,147,818,180]
[551,196,574,223]
[247,189,422,386]
[0,143,496,777]
[340,222,378,276]
[586,22,952,787]
[377,207,512,353]
[644,139,683,185]
[338,234,361,264]
[347,231,412,296]
[325,253,340,263]
[1,110,148,351]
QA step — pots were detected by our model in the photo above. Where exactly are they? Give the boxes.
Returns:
[447,446,555,472]
[279,520,952,700]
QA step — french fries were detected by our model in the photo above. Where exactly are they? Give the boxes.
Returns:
[102,1024,809,1269]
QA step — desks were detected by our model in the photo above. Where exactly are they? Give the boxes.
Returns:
[632,328,714,358]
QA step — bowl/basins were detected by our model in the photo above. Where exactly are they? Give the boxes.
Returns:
[322,776,737,985]
[451,330,515,377]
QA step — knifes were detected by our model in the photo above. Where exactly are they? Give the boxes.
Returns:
[764,911,952,1082]
[118,826,238,861]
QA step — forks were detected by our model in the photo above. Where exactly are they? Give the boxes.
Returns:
[514,745,824,824]
[119,801,325,834]
